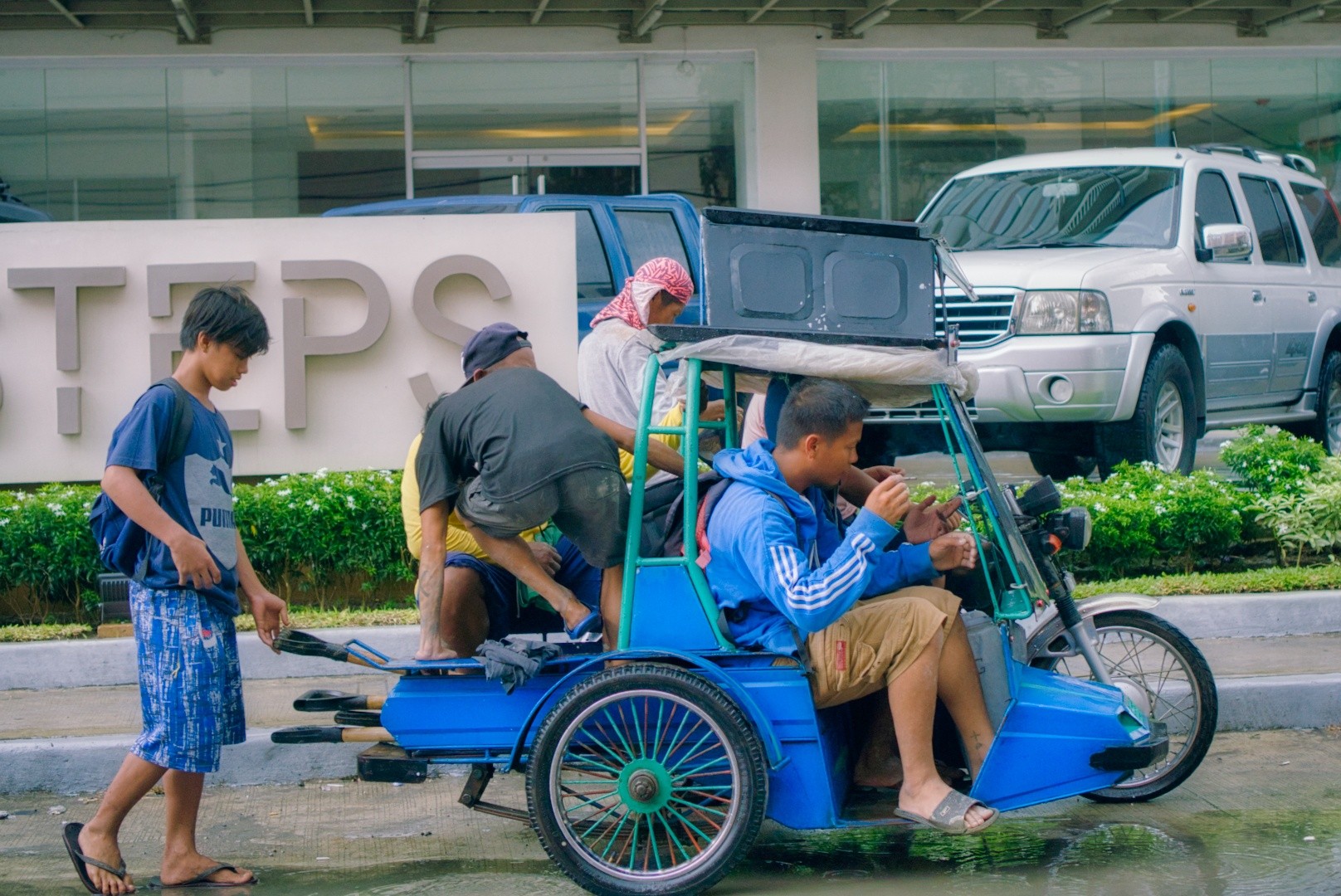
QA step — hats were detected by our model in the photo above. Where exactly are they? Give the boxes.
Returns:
[458,321,532,389]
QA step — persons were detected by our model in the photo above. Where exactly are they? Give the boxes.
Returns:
[701,380,997,834]
[62,286,290,896]
[400,259,961,793]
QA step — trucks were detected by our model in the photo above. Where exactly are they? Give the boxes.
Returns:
[322,197,753,412]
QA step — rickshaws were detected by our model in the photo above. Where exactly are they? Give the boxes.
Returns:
[378,334,1218,896]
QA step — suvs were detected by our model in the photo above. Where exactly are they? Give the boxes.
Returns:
[855,141,1341,474]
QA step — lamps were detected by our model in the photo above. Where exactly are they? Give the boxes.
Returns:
[97,572,132,627]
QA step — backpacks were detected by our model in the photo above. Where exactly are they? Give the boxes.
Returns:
[646,470,810,673]
[89,377,195,585]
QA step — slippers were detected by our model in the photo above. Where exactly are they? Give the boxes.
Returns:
[562,601,602,639]
[857,759,966,794]
[892,788,999,835]
[61,820,138,893]
[149,860,257,888]
